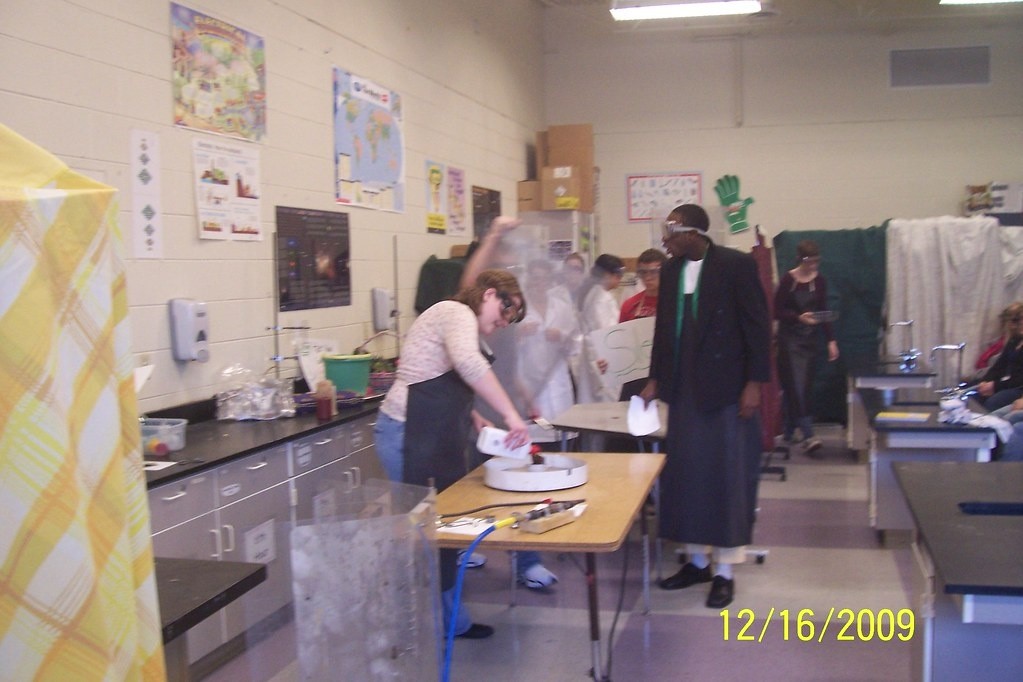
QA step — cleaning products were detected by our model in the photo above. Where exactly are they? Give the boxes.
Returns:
[476,424,541,460]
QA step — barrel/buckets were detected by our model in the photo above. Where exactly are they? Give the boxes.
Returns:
[322,353,374,396]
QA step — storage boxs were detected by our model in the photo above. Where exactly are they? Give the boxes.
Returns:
[518,122,595,214]
[140,418,188,456]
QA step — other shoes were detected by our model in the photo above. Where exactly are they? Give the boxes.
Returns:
[516,564,558,587]
[660,563,711,589]
[456,550,485,567]
[455,624,494,638]
[801,436,822,454]
[707,575,732,609]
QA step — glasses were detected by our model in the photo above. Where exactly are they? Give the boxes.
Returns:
[663,221,705,239]
[637,266,660,275]
[502,297,519,323]
[616,266,626,276]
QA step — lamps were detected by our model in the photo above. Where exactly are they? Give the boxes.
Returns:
[609,0,762,22]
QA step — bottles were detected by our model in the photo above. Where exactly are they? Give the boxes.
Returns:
[476,426,541,461]
[315,380,333,423]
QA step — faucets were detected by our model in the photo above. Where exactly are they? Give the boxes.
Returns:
[930,343,966,399]
[888,320,917,359]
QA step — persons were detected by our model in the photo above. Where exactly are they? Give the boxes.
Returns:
[372,268,532,640]
[454,213,671,592]
[962,302,1023,463]
[637,203,774,608]
[776,239,839,456]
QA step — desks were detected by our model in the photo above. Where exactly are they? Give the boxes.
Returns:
[890,459,1023,682]
[855,388,996,548]
[418,452,666,682]
[551,400,668,575]
[842,354,936,459]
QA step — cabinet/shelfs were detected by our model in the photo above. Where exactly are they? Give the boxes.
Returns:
[145,409,391,665]
